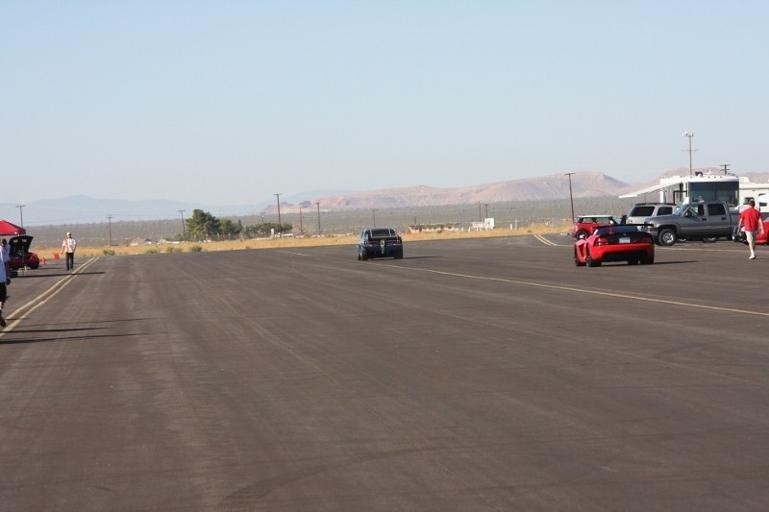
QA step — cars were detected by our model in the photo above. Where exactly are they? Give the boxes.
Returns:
[354,228,404,261]
[5,234,40,270]
[562,198,768,269]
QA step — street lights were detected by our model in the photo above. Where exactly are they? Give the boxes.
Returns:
[104,216,115,246]
[16,204,27,228]
[684,130,695,177]
[177,209,186,234]
[274,193,284,236]
[565,173,576,224]
[316,202,321,234]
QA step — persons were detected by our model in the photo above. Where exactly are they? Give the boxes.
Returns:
[738,199,765,260]
[0,241,8,333]
[61,232,77,271]
[1,238,12,280]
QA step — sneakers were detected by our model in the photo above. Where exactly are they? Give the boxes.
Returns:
[0,316,5,328]
